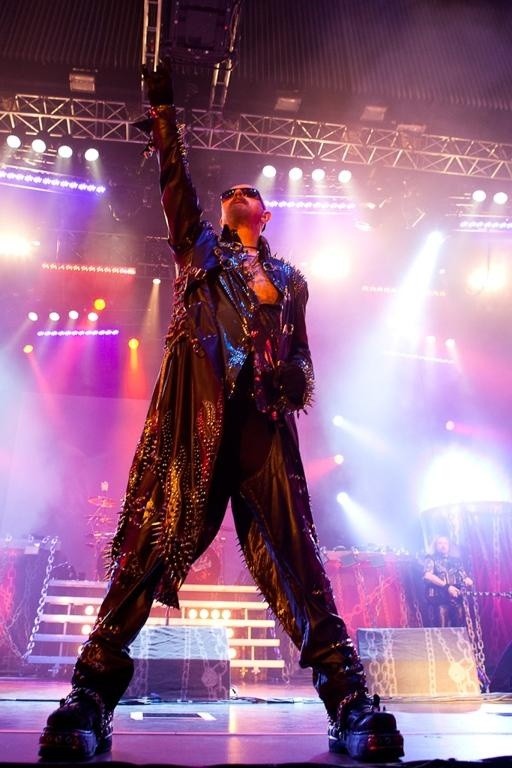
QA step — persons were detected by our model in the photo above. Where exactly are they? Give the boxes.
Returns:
[36,56,408,762]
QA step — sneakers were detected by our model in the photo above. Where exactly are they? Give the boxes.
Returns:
[325,688,404,761]
[38,689,114,760]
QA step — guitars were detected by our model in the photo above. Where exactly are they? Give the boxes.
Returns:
[425,582,512,606]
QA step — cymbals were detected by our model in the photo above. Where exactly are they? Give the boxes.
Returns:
[85,514,106,519]
[98,517,120,523]
[220,525,234,533]
[87,495,119,509]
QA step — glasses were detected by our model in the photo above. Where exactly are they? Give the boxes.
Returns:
[218,188,265,208]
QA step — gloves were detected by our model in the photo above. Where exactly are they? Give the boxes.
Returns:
[141,60,173,105]
[262,360,306,411]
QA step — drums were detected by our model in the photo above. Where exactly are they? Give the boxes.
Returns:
[183,547,221,584]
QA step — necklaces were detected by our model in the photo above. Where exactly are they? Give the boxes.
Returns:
[240,241,267,287]
